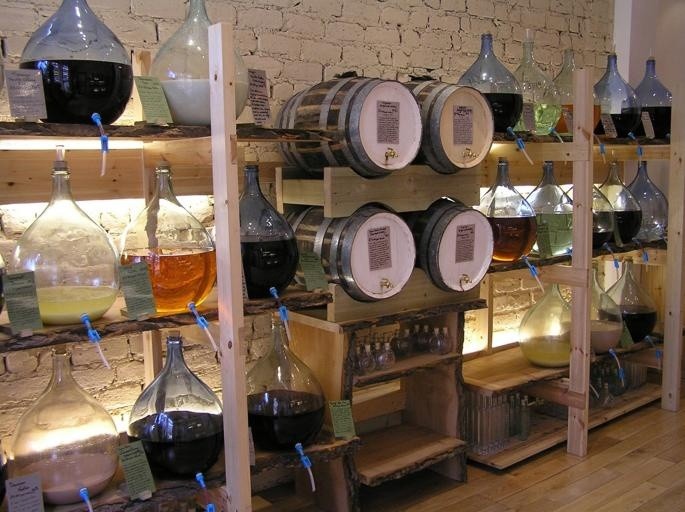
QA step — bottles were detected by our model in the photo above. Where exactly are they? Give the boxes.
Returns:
[596,257,658,343]
[238,162,298,300]
[596,158,643,245]
[10,144,120,325]
[122,158,217,314]
[520,279,573,367]
[521,160,575,256]
[460,389,535,454]
[20,0,132,125]
[353,324,452,374]
[8,345,120,506]
[511,27,564,137]
[560,176,614,257]
[617,161,669,243]
[633,56,673,141]
[478,157,540,262]
[153,0,250,126]
[545,45,604,137]
[248,312,326,454]
[592,53,641,143]
[588,263,625,356]
[460,33,523,137]
[589,360,649,409]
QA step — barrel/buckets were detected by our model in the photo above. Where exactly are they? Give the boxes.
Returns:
[399,198,495,293]
[286,206,417,301]
[399,77,495,176]
[275,73,421,179]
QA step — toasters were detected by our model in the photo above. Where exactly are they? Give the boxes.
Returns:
[127,330,224,481]
